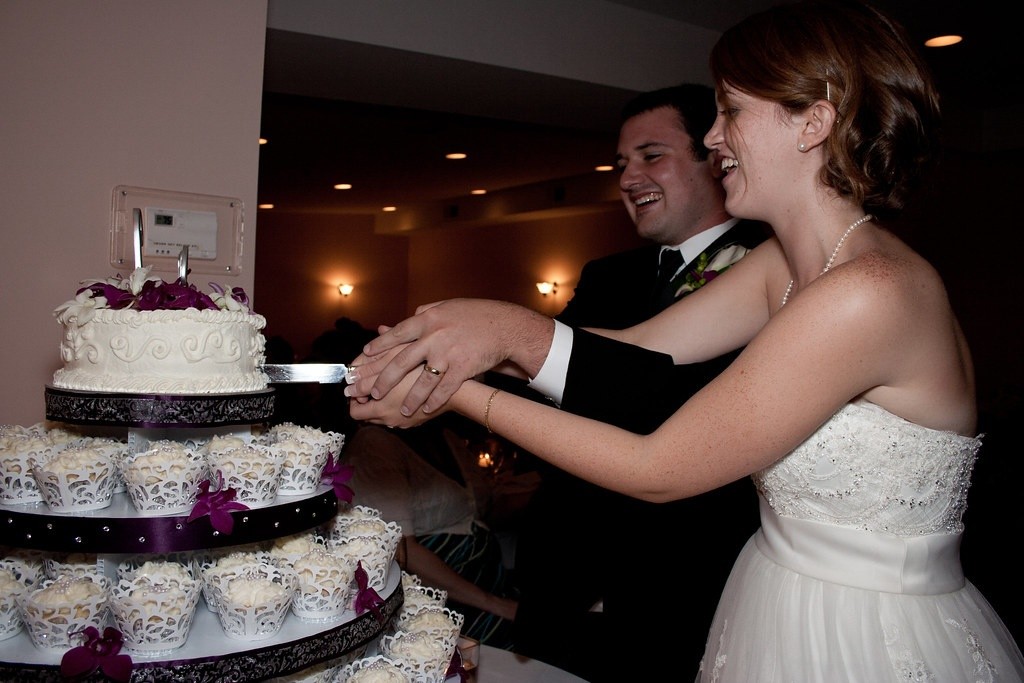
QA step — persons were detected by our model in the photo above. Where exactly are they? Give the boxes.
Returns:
[343,1,1024,683]
[266,319,549,622]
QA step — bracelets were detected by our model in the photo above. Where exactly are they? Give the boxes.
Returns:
[484,389,500,433]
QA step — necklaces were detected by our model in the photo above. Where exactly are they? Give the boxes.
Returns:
[779,214,872,310]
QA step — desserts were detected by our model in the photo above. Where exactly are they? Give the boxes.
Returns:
[259,572,464,683]
[1,500,403,657]
[0,417,345,516]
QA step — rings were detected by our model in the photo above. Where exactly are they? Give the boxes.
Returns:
[423,365,444,377]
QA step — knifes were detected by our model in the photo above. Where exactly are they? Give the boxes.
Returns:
[262,364,361,385]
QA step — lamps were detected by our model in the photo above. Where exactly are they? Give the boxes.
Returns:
[338,284,354,297]
[536,281,557,297]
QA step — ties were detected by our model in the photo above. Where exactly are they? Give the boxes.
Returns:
[657,248,685,286]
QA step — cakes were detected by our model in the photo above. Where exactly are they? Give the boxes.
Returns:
[53,265,272,398]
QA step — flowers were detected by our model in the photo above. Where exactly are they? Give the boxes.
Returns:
[187,469,250,536]
[351,561,385,625]
[53,289,111,327]
[318,451,356,504]
[60,626,132,683]
[685,251,730,292]
[77,263,256,316]
